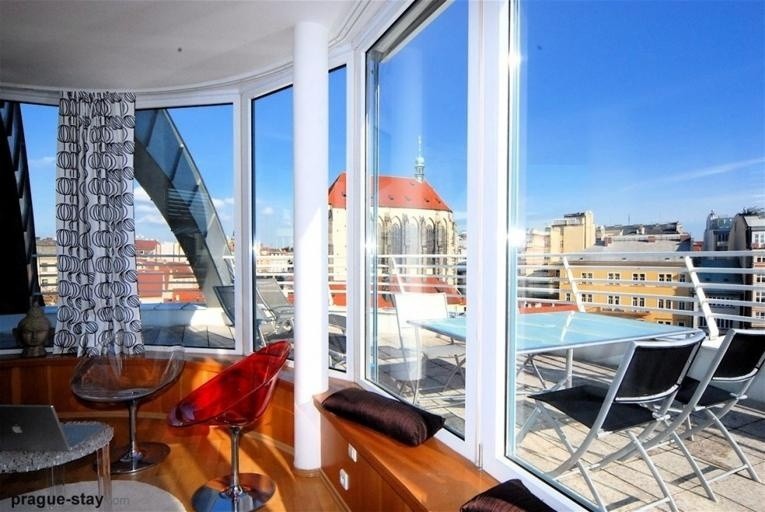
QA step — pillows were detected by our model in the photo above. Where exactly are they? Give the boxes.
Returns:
[320,384,441,448]
[461,479,551,512]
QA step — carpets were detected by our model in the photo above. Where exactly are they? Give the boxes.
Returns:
[1,477,188,512]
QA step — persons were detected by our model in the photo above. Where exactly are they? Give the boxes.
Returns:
[11,301,51,358]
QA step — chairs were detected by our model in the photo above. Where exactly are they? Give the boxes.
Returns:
[68,323,189,473]
[512,330,706,512]
[163,338,291,512]
[606,328,765,503]
[390,290,469,403]
[211,276,347,368]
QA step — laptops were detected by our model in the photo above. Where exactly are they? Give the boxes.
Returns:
[0,405,103,451]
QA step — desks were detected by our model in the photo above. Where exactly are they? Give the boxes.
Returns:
[406,309,700,450]
[1,415,118,511]
